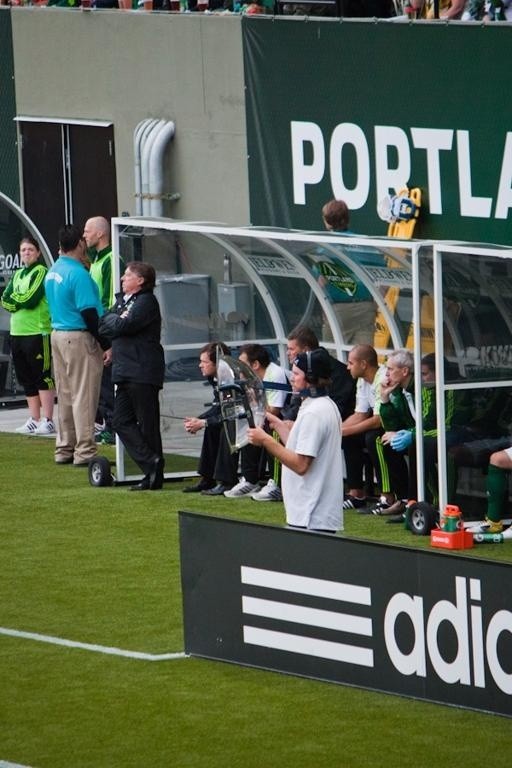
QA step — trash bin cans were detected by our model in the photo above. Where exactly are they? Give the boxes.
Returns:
[154,273,211,364]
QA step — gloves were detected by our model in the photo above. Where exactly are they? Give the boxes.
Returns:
[391,430,414,452]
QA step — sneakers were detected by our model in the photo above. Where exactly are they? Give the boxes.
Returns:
[500,525,512,540]
[93,423,116,445]
[342,494,407,523]
[17,417,39,433]
[253,479,283,502]
[466,517,503,534]
[56,458,88,466]
[225,477,262,497]
[35,418,53,435]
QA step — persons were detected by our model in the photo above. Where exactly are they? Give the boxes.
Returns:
[0,236,57,436]
[312,200,381,347]
[44,224,113,470]
[183,314,512,538]
[1,0,510,23]
[56,232,89,270]
[82,216,114,445]
[93,260,165,491]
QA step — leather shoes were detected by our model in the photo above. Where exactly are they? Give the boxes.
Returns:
[149,457,165,490]
[183,479,216,493]
[201,483,234,496]
[128,475,150,492]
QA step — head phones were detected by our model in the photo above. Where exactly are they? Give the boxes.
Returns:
[306,352,319,385]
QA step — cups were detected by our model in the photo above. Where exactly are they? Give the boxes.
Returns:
[442,504,462,531]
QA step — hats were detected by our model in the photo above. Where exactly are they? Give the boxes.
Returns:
[294,349,331,378]
